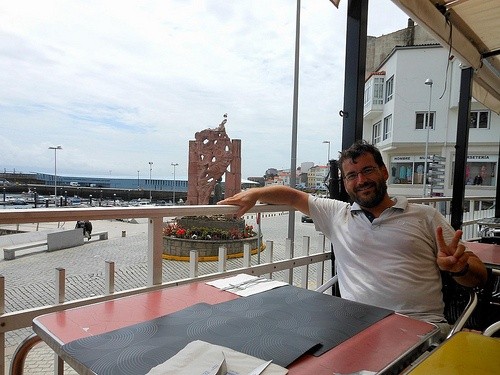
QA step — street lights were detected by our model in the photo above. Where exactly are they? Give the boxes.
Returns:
[422,78,433,198]
[148,162,154,203]
[323,141,330,161]
[170,163,179,206]
[47,145,63,207]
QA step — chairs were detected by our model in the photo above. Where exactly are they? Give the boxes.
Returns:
[314,273,477,375]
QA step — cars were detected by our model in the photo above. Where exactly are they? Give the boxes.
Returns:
[129,199,151,206]
[37,195,129,207]
[301,214,313,223]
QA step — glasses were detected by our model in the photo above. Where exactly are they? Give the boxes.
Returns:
[344,167,380,180]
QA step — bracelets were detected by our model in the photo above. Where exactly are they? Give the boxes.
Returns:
[449,263,469,277]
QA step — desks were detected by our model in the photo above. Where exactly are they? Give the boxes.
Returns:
[8,218,500,375]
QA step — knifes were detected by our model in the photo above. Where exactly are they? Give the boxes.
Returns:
[246,359,273,375]
[219,275,264,291]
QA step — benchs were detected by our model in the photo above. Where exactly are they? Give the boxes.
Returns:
[3,231,108,260]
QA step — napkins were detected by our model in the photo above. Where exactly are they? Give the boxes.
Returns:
[145,339,288,375]
[205,273,289,298]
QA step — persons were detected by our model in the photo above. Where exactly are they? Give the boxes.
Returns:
[473,174,483,185]
[217,140,487,342]
[75,220,92,241]
[399,165,411,181]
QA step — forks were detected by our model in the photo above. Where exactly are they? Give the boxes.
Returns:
[213,351,228,375]
[228,279,273,290]
